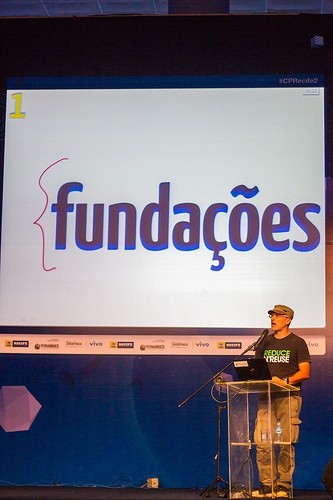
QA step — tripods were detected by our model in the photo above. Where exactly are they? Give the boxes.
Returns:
[178,341,256,496]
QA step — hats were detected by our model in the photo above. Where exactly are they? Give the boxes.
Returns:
[268,305,294,319]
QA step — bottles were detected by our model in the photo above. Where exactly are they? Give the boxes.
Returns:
[276,419,283,442]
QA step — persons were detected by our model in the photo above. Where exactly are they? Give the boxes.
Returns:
[248,305,313,499]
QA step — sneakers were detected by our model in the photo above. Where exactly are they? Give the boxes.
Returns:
[276,485,290,498]
[251,485,276,497]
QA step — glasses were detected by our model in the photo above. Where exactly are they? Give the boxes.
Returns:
[269,314,289,318]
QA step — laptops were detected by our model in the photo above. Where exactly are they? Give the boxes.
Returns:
[234,358,272,381]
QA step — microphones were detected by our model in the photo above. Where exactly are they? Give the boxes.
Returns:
[253,329,269,352]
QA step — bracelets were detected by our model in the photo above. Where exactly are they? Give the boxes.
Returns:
[286,377,289,383]
